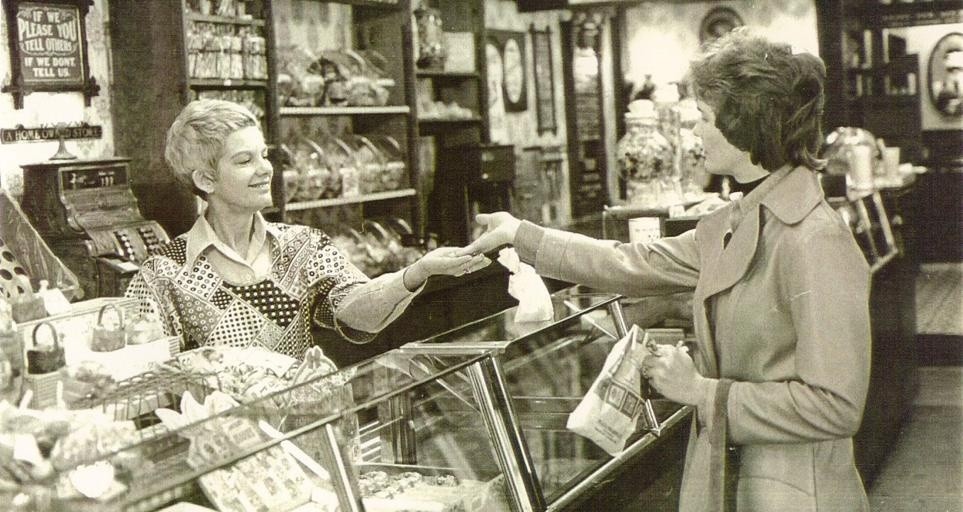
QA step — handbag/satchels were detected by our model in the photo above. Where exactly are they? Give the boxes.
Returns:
[27,322,65,374]
[91,305,125,352]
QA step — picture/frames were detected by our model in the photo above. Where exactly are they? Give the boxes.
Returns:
[4,1,96,112]
[924,32,963,117]
[697,5,745,53]
[484,23,558,137]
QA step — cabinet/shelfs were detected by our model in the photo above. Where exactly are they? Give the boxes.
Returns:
[105,1,521,368]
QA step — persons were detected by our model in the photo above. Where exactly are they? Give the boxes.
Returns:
[455,21,874,512]
[123,98,492,367]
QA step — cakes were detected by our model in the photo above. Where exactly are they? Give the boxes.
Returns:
[432,473,458,487]
[357,470,421,499]
